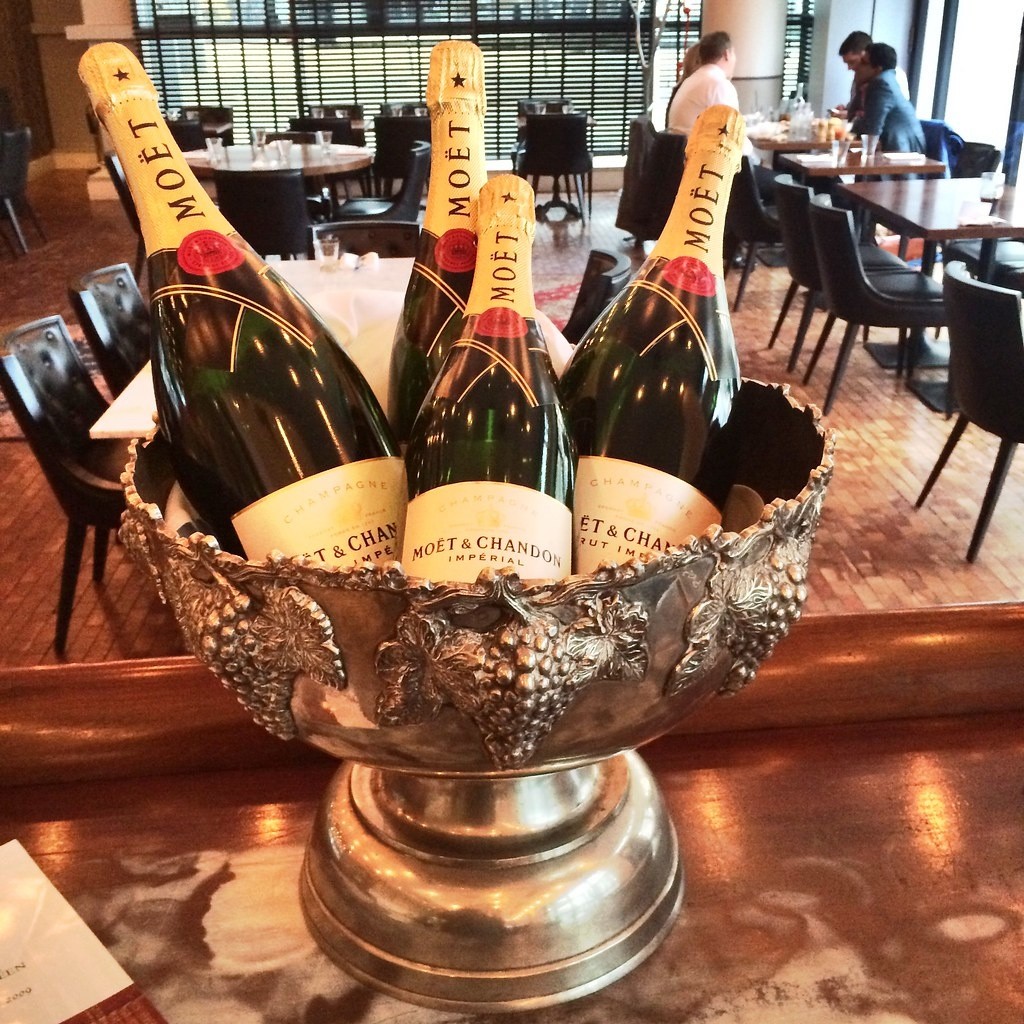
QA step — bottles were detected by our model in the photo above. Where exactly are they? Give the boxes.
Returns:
[387,38,488,456]
[395,171,574,583]
[76,41,406,568]
[558,102,745,573]
[791,83,807,141]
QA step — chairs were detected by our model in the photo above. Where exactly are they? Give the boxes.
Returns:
[916,260,1024,564]
[920,119,1024,299]
[0,126,48,253]
[724,155,954,422]
[561,249,632,346]
[615,118,688,248]
[0,315,125,657]
[66,262,150,398]
[85,103,433,260]
[518,99,593,227]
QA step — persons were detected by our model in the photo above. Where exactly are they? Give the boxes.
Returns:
[832,31,927,185]
[664,28,785,268]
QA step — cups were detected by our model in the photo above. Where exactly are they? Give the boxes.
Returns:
[334,110,351,120]
[832,139,851,163]
[533,100,547,115]
[414,108,427,117]
[165,107,179,124]
[861,134,880,155]
[390,103,404,117]
[205,126,335,166]
[311,106,323,121]
[311,236,341,274]
[563,103,573,116]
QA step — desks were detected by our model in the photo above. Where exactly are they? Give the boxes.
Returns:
[782,152,946,311]
[835,178,1024,369]
[201,121,233,136]
[2,601,1023,1023]
[749,134,882,267]
[516,115,596,222]
[90,257,416,439]
[323,119,376,132]
[181,144,373,177]
